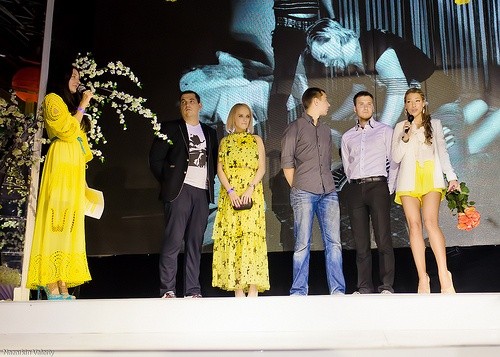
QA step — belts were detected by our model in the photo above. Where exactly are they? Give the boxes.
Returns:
[351,177,381,184]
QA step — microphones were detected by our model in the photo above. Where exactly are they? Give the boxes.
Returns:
[77,85,97,106]
[405,115,414,134]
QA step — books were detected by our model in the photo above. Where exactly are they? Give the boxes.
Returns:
[85,187,104,220]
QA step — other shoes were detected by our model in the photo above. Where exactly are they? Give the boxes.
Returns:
[163,290,204,299]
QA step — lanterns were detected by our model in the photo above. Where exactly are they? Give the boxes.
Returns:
[12,66,41,113]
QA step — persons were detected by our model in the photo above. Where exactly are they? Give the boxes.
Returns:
[280,87,345,296]
[341,91,400,295]
[391,88,462,293]
[264,0,500,156]
[148,90,218,298]
[23,64,94,300]
[211,103,270,297]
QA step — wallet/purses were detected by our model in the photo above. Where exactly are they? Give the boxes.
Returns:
[232,198,252,210]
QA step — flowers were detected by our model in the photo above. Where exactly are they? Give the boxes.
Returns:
[443,173,481,232]
[0,53,174,257]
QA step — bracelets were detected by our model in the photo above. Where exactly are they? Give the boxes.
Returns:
[227,188,234,194]
[77,107,85,114]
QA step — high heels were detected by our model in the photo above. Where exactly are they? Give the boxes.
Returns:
[441,271,456,293]
[34,280,76,300]
[418,275,430,294]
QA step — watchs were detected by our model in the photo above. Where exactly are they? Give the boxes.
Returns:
[248,183,255,190]
